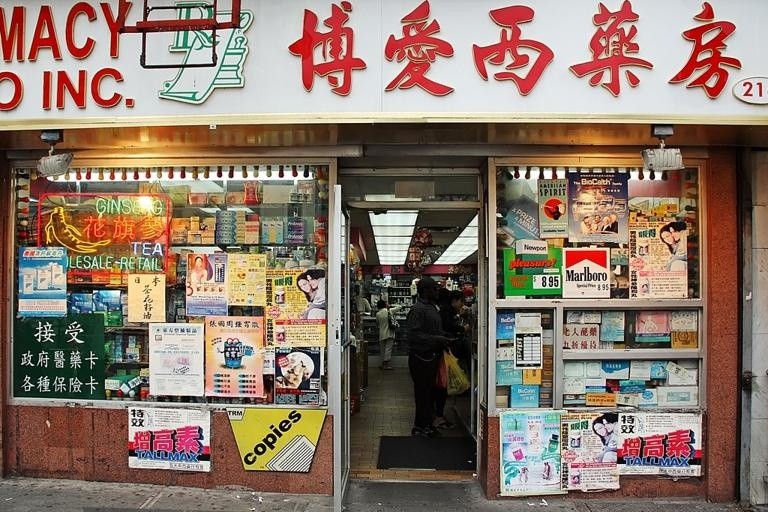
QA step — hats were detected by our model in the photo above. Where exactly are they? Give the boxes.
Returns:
[416,278,441,292]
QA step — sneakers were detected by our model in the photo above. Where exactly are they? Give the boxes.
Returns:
[411,425,442,440]
[432,416,456,430]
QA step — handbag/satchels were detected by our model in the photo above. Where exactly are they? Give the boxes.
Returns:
[388,314,400,329]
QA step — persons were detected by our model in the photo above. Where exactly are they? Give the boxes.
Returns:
[406,278,471,440]
[190,257,208,284]
[660,222,687,270]
[582,214,618,235]
[296,269,326,320]
[375,299,402,370]
[356,296,372,312]
[592,413,617,463]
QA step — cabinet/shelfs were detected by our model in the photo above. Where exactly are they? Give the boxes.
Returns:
[491,303,705,414]
[102,170,314,402]
[361,279,418,318]
